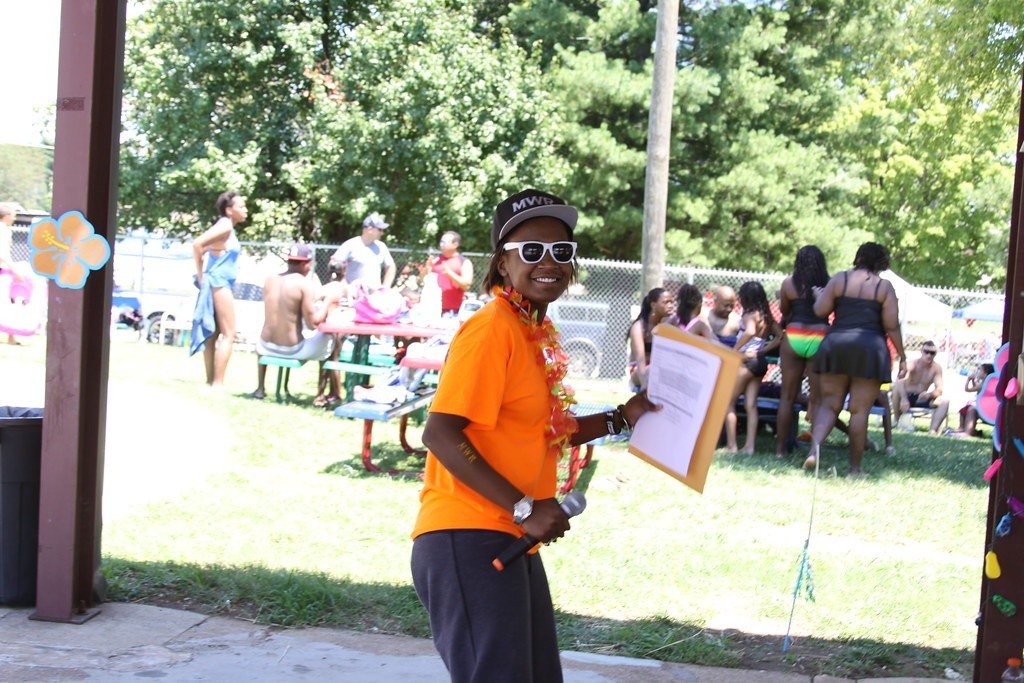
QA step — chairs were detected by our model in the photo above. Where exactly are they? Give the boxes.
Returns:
[891,366,951,433]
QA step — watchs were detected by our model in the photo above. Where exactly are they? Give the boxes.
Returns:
[513,496,534,525]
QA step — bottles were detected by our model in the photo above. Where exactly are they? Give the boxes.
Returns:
[956,367,977,377]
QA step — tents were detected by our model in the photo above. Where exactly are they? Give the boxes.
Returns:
[880,269,950,370]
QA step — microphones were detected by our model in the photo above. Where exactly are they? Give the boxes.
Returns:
[493,491,587,572]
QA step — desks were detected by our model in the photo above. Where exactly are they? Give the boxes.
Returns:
[320,312,463,401]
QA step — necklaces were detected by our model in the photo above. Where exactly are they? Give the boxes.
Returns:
[492,284,579,459]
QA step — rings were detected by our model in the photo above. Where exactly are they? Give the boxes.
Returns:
[545,539,555,546]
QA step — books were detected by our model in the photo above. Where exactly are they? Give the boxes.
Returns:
[628,324,744,494]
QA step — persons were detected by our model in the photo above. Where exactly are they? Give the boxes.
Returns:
[724,280,783,453]
[625,284,741,390]
[802,242,907,474]
[954,364,994,436]
[0,206,21,343]
[411,189,663,683]
[776,245,831,457]
[420,231,473,317]
[892,340,949,434]
[835,393,893,452]
[254,245,355,407]
[190,191,248,387]
[327,212,396,288]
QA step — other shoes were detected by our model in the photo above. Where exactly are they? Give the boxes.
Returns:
[886,446,896,459]
[868,437,879,453]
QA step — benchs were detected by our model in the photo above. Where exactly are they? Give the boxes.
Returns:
[332,379,439,480]
[732,394,801,451]
[260,354,307,403]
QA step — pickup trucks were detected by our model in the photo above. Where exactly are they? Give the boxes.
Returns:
[545,300,610,380]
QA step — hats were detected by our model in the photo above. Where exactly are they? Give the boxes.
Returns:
[285,244,313,262]
[363,211,389,230]
[490,189,578,251]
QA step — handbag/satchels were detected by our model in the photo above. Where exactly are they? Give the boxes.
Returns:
[355,283,402,325]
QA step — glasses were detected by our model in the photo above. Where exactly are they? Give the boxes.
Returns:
[922,348,936,356]
[499,241,577,264]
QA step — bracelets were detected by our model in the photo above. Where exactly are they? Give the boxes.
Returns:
[606,411,621,435]
[617,404,632,430]
